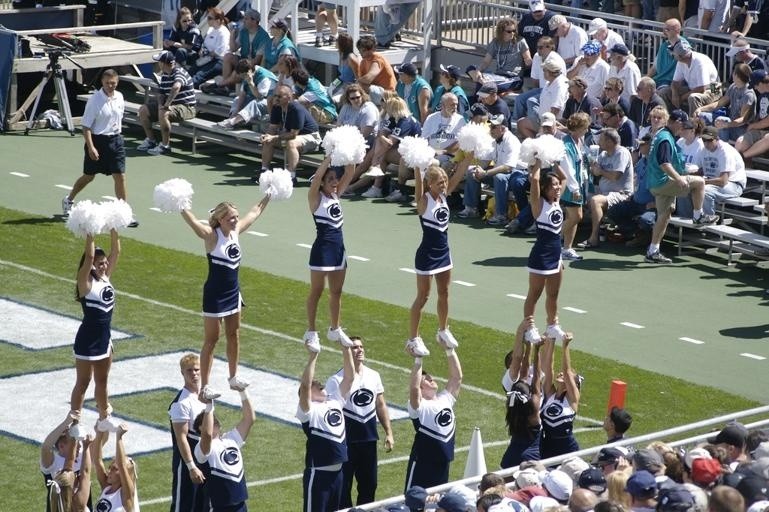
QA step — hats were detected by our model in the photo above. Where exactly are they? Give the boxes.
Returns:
[150,0,769,136]
[389,421,769,512]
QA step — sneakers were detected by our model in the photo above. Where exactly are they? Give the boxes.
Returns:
[69,423,87,441]
[457,207,540,235]
[137,137,171,156]
[303,326,322,355]
[95,419,120,434]
[435,327,459,350]
[199,384,221,400]
[692,212,719,226]
[405,335,431,358]
[62,194,73,217]
[524,326,544,344]
[360,184,404,202]
[546,323,569,341]
[326,326,354,348]
[227,375,250,391]
[562,240,672,265]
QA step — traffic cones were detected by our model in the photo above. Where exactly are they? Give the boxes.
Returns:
[462,426,487,492]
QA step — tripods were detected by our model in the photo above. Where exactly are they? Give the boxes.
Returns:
[24,58,76,137]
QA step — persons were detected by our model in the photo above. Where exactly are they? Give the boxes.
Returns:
[353,406,769,511]
[501,135,582,469]
[63,69,139,227]
[139,1,338,185]
[331,2,768,263]
[396,119,494,493]
[296,127,394,511]
[39,197,139,511]
[151,168,294,510]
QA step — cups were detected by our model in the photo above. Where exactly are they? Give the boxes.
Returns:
[589,144,601,161]
